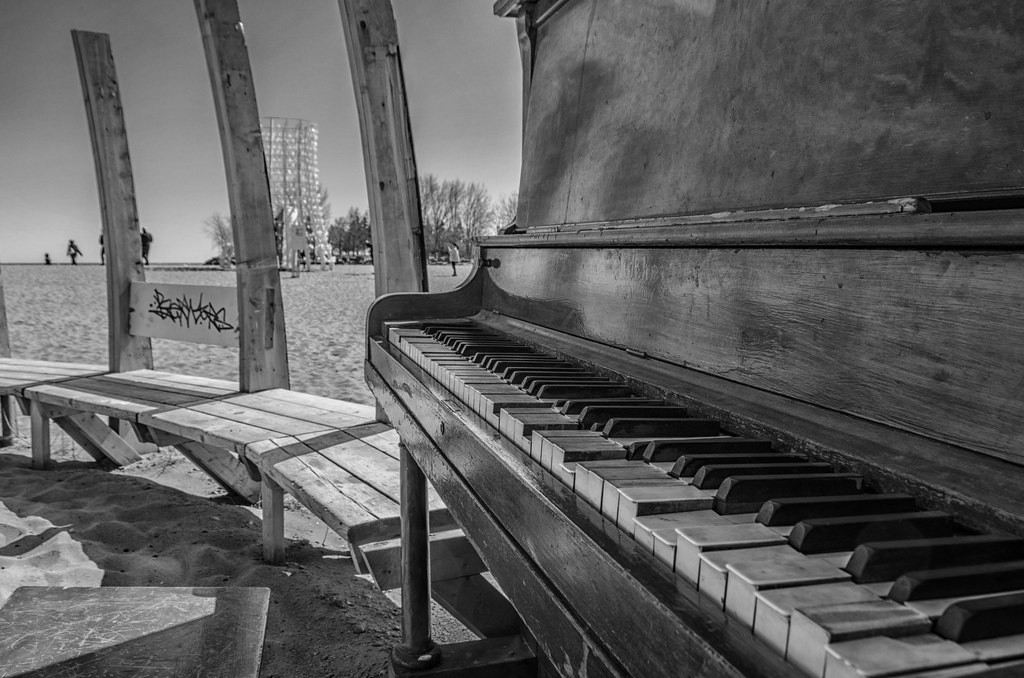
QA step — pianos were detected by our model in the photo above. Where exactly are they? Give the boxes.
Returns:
[362,0,1024,678]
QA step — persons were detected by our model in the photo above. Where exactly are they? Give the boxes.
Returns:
[66,240,83,265]
[99,231,105,265]
[140,227,152,265]
[44,252,51,266]
[447,243,460,276]
[366,238,375,274]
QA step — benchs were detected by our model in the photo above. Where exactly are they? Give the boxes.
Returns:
[0,358,523,639]
[0,586,270,678]
